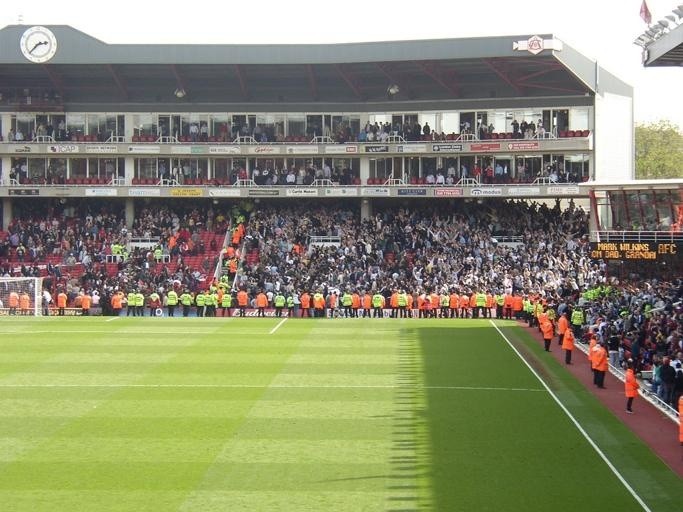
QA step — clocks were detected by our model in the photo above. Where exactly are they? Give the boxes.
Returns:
[20,26,57,64]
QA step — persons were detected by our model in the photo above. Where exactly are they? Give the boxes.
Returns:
[539,318,553,353]
[556,311,569,346]
[0,113,592,321]
[586,333,598,375]
[561,323,577,365]
[677,395,682,448]
[592,211,682,419]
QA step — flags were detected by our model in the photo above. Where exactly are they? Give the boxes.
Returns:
[638,0,652,27]
